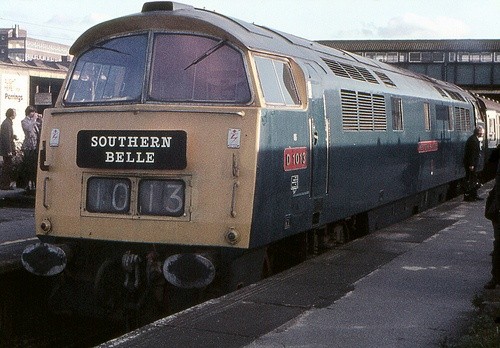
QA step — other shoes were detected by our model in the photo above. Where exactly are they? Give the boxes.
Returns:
[464,197,477,202]
[476,196,484,201]
[484,278,500,290]
[26,185,35,191]
[0,185,14,190]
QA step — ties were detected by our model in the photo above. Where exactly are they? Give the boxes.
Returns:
[34,124,39,148]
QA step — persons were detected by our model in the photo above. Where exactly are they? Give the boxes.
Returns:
[20,106,43,192]
[463,143,500,290]
[462,125,485,201]
[0,108,19,190]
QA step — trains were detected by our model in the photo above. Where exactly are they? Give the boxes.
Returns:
[20,1,500,288]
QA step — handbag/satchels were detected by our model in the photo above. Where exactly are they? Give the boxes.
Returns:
[461,169,481,194]
